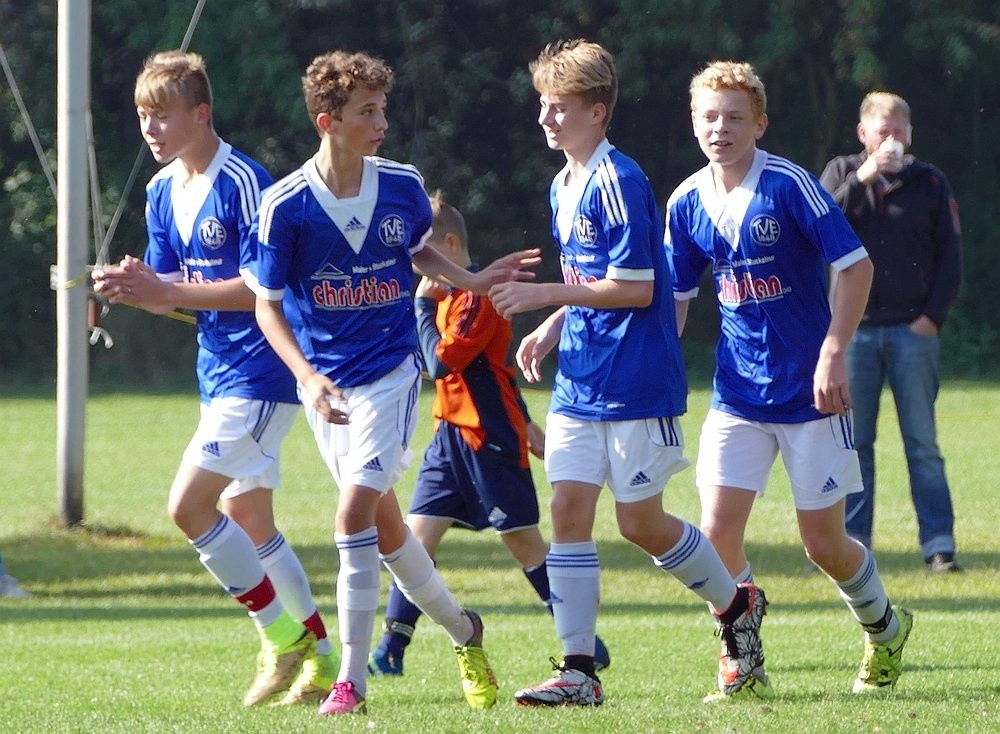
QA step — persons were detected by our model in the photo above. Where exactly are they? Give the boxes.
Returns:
[244,50,543,713]
[664,60,913,704]
[367,189,613,677]
[818,91,966,573]
[92,49,342,707]
[488,38,769,707]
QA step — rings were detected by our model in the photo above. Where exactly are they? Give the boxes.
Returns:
[128,286,132,293]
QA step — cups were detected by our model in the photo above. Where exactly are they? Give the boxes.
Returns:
[879,140,904,170]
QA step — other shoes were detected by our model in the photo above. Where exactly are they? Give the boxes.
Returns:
[929,553,958,572]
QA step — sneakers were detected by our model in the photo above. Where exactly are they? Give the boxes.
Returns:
[319,681,368,716]
[853,605,913,694]
[453,608,499,708]
[366,652,403,676]
[742,674,774,700]
[270,649,341,708]
[706,583,768,695]
[514,656,605,708]
[593,636,609,670]
[242,619,316,707]
